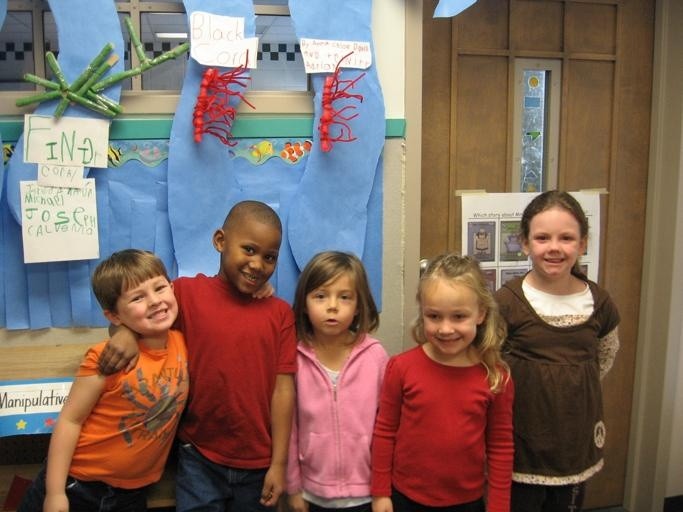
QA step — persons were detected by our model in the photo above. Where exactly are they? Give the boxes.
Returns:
[284,247,392,511]
[489,188,621,509]
[94,198,303,511]
[365,247,516,511]
[14,248,191,510]
